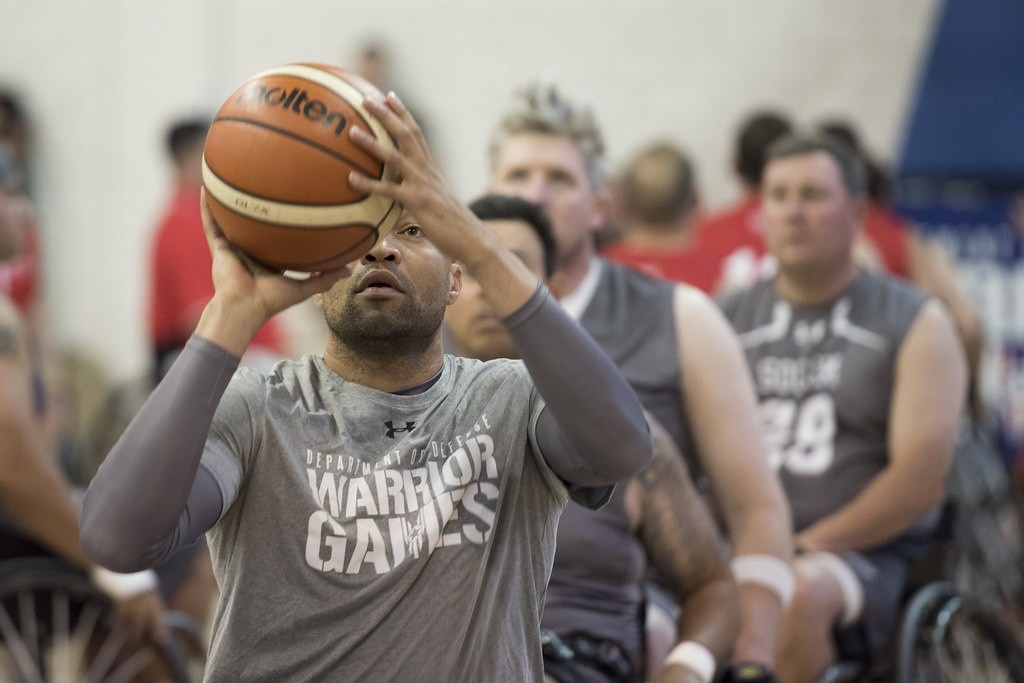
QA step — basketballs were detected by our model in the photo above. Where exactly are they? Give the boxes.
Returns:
[199,58,405,277]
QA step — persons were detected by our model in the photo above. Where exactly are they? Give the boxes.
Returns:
[0,78,981,683]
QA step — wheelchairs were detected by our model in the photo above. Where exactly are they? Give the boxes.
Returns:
[646,571,1024,682]
[0,530,214,683]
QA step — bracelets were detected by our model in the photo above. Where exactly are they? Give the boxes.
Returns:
[91,567,157,600]
[730,553,796,613]
[664,641,717,683]
[723,661,776,683]
